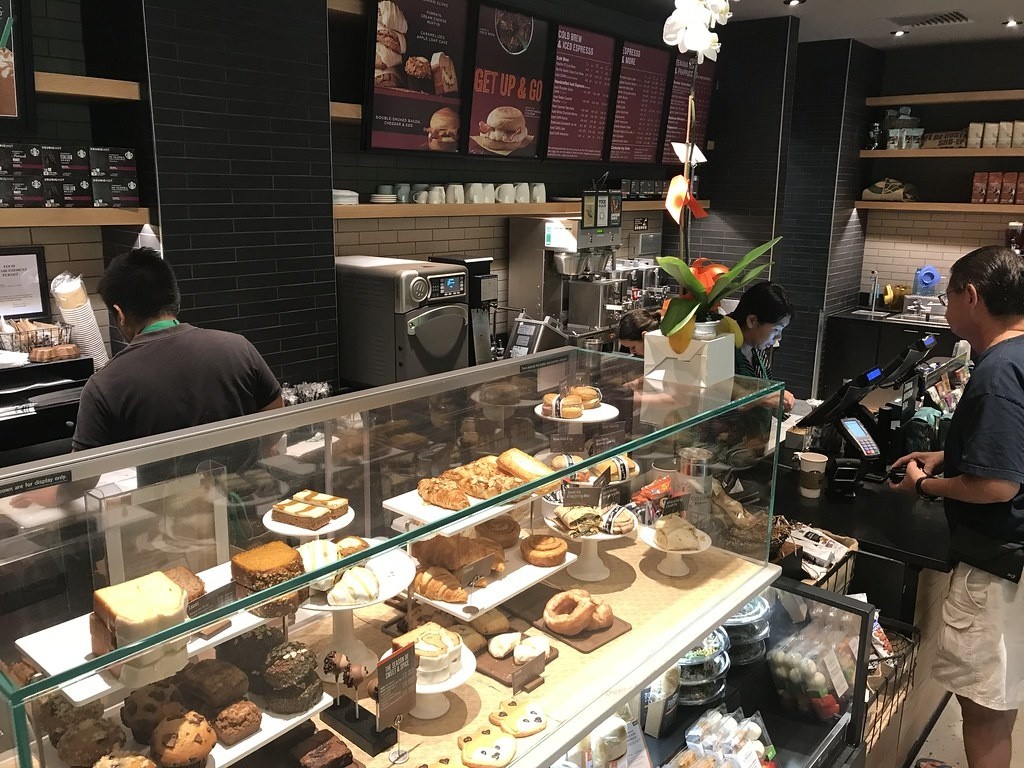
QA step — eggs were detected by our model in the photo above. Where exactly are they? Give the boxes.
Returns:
[770,650,825,689]
[707,711,764,760]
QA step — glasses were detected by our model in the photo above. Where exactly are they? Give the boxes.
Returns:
[938,282,979,307]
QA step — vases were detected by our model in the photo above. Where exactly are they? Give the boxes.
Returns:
[692,321,721,341]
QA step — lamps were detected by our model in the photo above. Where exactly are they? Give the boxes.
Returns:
[655,0,784,353]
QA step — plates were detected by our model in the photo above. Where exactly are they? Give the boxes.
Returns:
[534,402,619,423]
[495,9,534,55]
[550,197,582,201]
[371,194,397,204]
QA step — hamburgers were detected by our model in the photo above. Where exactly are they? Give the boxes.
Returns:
[479,106,527,151]
[426,107,460,152]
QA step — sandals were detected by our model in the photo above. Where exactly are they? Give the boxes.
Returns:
[916,758,956,768]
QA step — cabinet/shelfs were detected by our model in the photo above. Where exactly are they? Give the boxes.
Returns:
[262,506,417,683]
[0,1,710,227]
[14,561,367,768]
[854,88,1024,214]
[817,318,977,401]
[534,401,641,582]
[381,488,632,687]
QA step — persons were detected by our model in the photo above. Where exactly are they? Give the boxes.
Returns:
[616,309,682,407]
[886,243,1024,768]
[11,248,285,571]
[692,282,795,461]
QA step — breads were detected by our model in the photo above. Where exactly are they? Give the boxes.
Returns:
[168,380,535,577]
[9,447,700,768]
[375,0,459,95]
[540,385,601,417]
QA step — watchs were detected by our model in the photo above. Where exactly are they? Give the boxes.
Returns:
[916,470,939,503]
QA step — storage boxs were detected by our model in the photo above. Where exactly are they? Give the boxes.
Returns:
[643,329,735,387]
[971,172,1024,205]
[966,121,1024,148]
[608,179,669,200]
[0,144,139,207]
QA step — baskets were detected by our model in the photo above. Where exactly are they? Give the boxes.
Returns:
[815,551,855,596]
[860,619,922,758]
[0,319,75,353]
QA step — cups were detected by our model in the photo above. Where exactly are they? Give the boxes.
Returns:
[56,281,109,372]
[378,183,546,204]
[799,452,828,499]
